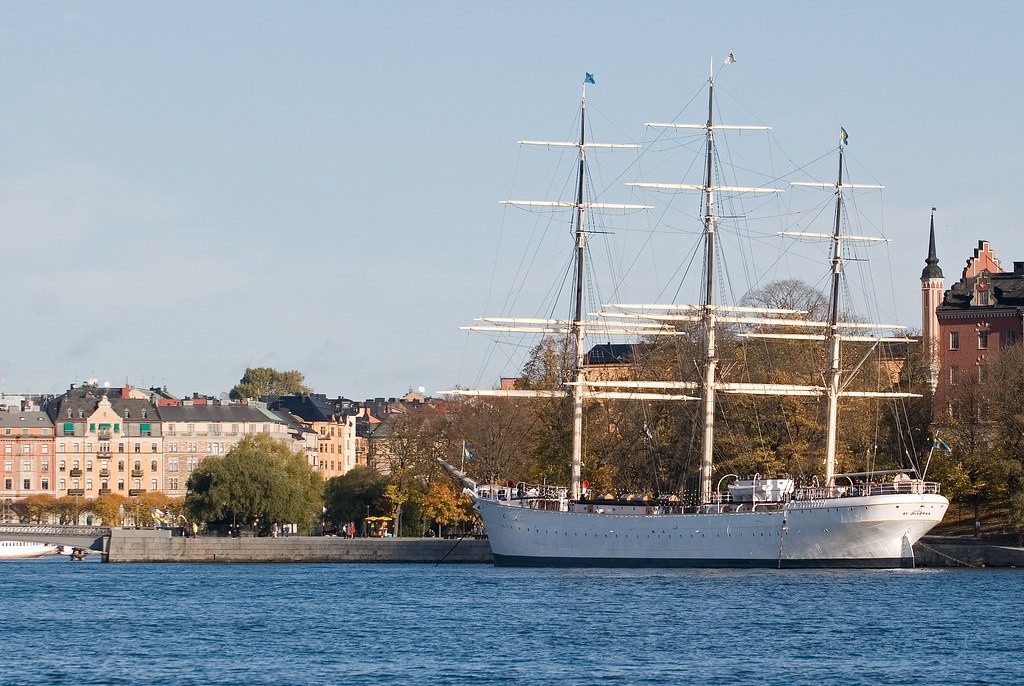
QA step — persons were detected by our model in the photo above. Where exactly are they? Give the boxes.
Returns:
[517,476,877,509]
[59,515,487,541]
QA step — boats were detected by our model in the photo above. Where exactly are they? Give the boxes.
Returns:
[0,539,59,558]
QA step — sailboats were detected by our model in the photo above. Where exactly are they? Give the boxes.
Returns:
[436,53,951,569]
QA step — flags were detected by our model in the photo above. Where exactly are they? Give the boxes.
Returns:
[933,436,951,452]
[464,442,475,462]
